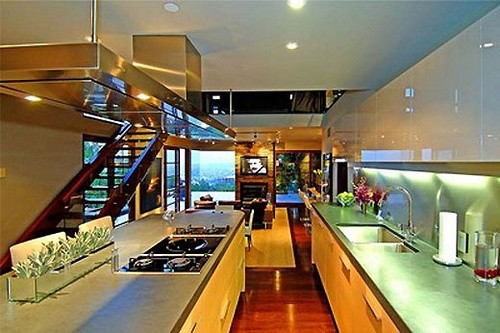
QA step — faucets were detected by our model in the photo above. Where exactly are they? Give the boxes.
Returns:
[377,186,420,240]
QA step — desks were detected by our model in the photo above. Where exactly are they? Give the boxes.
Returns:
[194,199,216,210]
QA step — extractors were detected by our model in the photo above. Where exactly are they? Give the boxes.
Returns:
[1,35,237,141]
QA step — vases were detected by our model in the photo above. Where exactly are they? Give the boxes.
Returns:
[361,200,367,214]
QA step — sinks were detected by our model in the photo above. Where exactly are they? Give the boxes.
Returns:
[336,220,409,245]
[350,242,421,256]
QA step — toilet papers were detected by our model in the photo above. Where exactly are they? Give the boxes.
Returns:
[438,210,457,264]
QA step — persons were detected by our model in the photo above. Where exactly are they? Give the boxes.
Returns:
[247,159,266,173]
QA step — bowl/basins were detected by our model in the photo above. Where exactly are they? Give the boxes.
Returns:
[335,196,356,206]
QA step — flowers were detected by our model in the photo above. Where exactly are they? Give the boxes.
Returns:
[353,179,371,202]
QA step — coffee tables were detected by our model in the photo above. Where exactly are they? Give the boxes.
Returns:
[249,204,274,231]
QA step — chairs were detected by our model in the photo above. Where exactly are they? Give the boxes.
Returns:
[243,200,268,226]
[244,209,255,250]
[214,205,234,211]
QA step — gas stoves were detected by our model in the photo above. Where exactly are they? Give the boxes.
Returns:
[113,224,232,275]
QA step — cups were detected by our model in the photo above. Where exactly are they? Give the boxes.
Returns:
[473,230,500,286]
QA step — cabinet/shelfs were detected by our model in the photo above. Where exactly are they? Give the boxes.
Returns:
[306,211,403,333]
[330,6,499,163]
[180,217,257,333]
